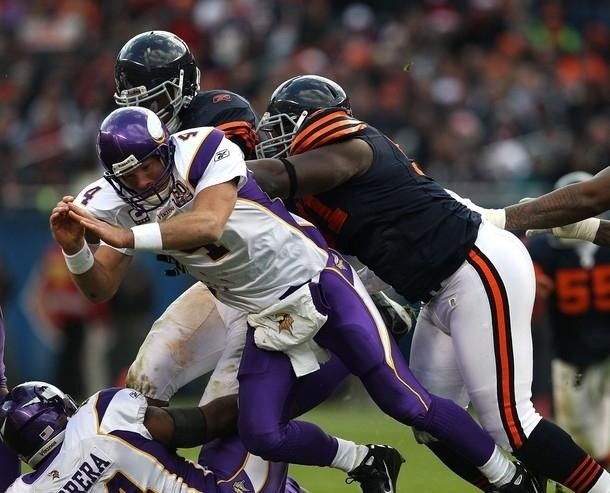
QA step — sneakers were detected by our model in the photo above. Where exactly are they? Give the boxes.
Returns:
[348,444,403,493]
[494,461,535,492]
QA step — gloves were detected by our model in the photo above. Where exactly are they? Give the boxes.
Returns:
[442,188,506,231]
[517,197,601,245]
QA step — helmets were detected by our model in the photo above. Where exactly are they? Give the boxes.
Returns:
[94,106,174,214]
[254,74,352,160]
[112,30,200,137]
[0,381,78,471]
